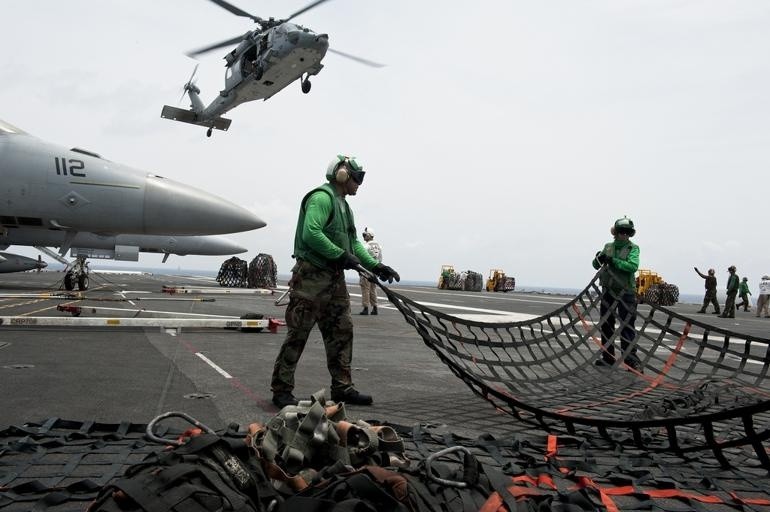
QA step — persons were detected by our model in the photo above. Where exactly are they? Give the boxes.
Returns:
[441,269,450,289]
[738,276,752,313]
[592,215,641,373]
[753,275,770,318]
[693,266,721,314]
[360,227,382,315]
[270,154,402,410]
[719,265,739,318]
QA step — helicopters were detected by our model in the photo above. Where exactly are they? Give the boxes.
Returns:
[160,0,380,137]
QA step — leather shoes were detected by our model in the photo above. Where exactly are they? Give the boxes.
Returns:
[624,358,643,373]
[331,389,373,405]
[273,394,298,409]
[596,357,616,366]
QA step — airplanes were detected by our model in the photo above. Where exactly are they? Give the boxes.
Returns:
[0,226,249,290]
[0,119,267,259]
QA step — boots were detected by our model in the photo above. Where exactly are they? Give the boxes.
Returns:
[360,307,368,315]
[371,306,377,315]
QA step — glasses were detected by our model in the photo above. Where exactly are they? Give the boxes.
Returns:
[346,167,365,185]
[619,227,635,236]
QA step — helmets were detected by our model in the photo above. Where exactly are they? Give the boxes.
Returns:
[326,155,362,181]
[615,218,634,229]
[363,227,373,237]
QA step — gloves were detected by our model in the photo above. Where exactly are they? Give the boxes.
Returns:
[596,251,614,267]
[336,249,361,270]
[371,263,400,283]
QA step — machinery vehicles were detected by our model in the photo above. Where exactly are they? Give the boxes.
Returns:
[636,269,665,304]
[438,265,455,289]
[485,269,505,291]
